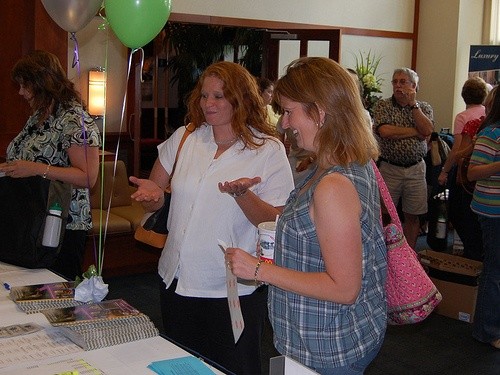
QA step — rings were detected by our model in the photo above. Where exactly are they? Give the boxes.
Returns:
[228,264,232,271]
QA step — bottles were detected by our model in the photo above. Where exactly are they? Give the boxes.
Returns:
[436,218,446,238]
[142,72,152,96]
[41,201,63,247]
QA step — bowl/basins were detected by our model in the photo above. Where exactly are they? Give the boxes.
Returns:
[158,59,168,65]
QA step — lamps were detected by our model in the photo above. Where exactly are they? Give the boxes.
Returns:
[86,65,109,120]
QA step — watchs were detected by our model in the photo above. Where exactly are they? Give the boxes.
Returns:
[410,102,419,110]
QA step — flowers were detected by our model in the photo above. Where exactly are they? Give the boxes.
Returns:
[346,48,387,117]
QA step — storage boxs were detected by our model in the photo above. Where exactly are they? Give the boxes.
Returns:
[418,250,483,323]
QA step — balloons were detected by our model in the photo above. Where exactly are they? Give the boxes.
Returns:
[104,0,173,51]
[42,0,103,34]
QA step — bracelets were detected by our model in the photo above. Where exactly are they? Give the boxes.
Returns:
[254,261,265,287]
[232,189,247,198]
[42,164,51,179]
[442,167,449,173]
[308,156,314,164]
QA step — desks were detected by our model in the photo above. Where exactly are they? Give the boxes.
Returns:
[0,262,226,375]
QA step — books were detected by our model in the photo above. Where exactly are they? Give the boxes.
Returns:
[8,281,160,351]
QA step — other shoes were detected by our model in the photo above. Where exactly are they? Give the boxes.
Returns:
[473,332,500,348]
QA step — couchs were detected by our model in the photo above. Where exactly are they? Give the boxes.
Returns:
[84,161,159,278]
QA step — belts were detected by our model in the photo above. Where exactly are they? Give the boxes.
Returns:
[380,157,422,168]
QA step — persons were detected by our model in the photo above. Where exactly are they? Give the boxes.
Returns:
[224,56,389,375]
[371,66,500,348]
[129,61,295,375]
[0,49,102,282]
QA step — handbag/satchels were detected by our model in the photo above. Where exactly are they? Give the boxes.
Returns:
[0,175,72,269]
[370,157,443,325]
[133,123,197,252]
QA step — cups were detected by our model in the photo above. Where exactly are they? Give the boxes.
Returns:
[258,220,277,266]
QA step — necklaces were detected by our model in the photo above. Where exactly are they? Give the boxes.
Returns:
[300,169,322,190]
